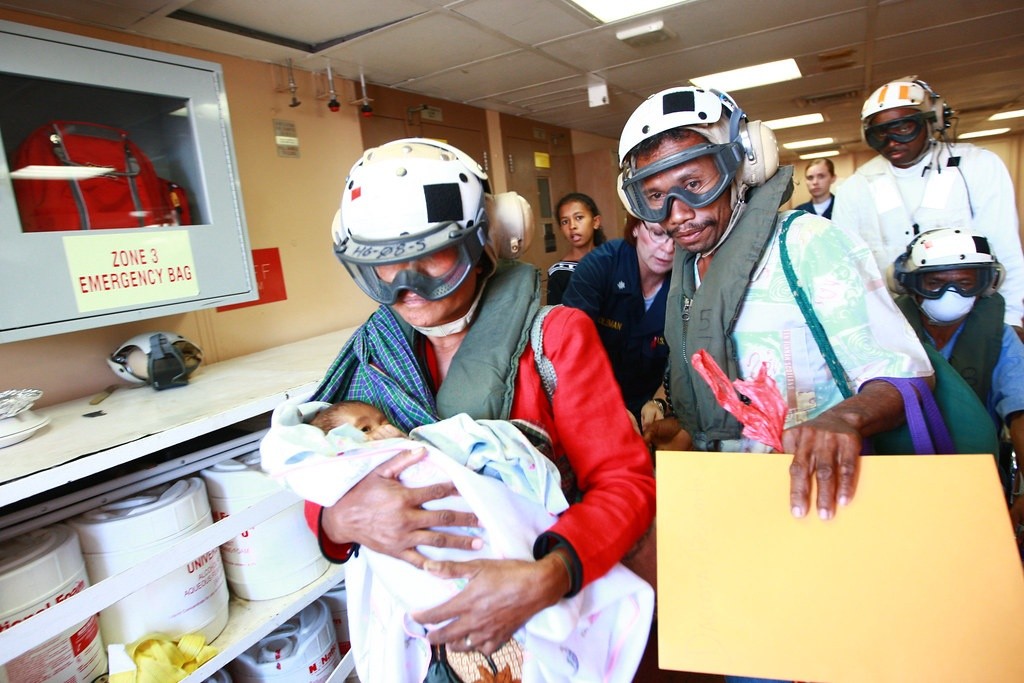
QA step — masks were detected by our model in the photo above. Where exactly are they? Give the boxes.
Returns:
[925,291,975,323]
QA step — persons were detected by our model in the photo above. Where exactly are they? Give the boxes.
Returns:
[885,228,1024,532]
[793,157,837,220]
[260,400,654,683]
[303,136,656,683]
[615,86,935,522]
[831,74,1024,344]
[561,212,675,469]
[547,192,607,306]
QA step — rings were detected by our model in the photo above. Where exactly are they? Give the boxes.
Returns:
[464,634,475,653]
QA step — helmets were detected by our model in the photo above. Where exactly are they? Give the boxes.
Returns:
[861,75,953,136]
[107,334,203,389]
[894,228,1007,297]
[617,86,780,222]
[330,139,536,306]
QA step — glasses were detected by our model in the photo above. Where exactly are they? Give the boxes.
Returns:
[640,220,674,244]
[865,111,928,150]
[334,223,480,304]
[898,264,992,300]
[621,136,748,222]
[148,351,189,389]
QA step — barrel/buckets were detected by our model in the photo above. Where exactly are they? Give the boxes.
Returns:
[0,449,359,683]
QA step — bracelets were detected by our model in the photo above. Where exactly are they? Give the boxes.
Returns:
[548,552,575,592]
[653,398,667,415]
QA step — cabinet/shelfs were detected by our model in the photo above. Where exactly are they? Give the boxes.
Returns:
[0,323,364,683]
